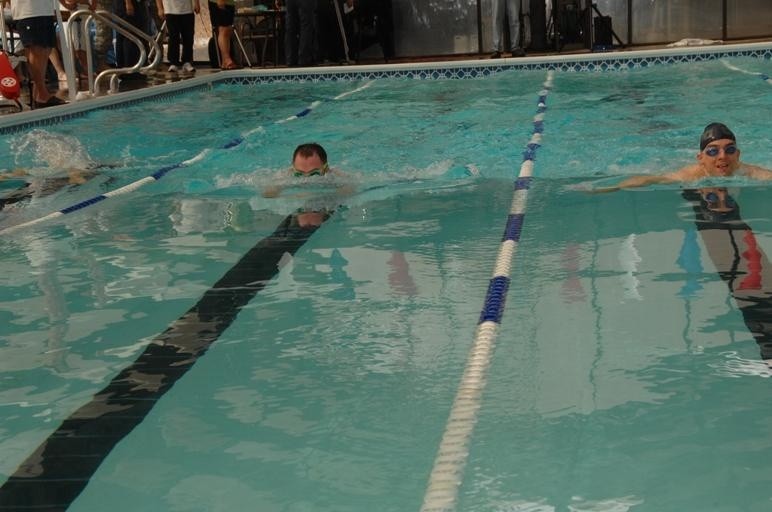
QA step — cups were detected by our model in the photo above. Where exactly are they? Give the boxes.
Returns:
[702,145,737,156]
[292,162,327,177]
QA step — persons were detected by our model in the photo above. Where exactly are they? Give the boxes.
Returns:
[0,0,397,109]
[297,210,326,231]
[0,162,127,185]
[491,0,525,58]
[697,187,737,222]
[262,143,354,199]
[566,122,772,195]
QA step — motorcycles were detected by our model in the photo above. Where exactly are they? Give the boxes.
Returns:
[547,0,626,50]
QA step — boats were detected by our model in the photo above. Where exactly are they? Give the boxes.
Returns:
[552,0,589,50]
[593,15,613,49]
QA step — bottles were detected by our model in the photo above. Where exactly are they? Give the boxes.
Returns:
[700,122,736,151]
